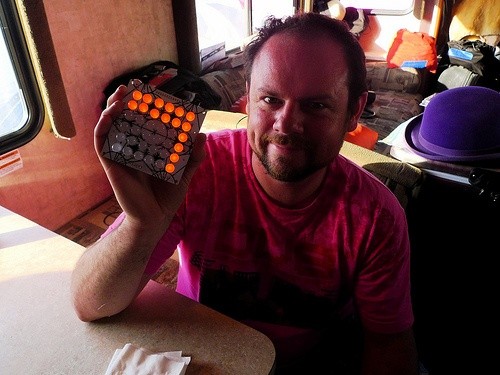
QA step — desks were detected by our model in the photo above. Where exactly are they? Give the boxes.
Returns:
[0,205,277,375]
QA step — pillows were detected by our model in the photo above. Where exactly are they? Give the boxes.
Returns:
[344,122,379,150]
[387,29,438,69]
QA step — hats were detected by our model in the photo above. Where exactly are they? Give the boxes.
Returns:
[405,86,500,163]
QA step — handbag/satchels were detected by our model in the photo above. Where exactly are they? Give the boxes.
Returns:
[387,29,438,73]
[441,34,496,76]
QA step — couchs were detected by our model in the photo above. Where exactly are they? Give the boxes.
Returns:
[198,61,424,157]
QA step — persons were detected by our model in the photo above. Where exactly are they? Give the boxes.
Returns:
[70,12,416,375]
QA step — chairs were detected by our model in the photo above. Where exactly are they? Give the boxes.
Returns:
[198,106,425,210]
